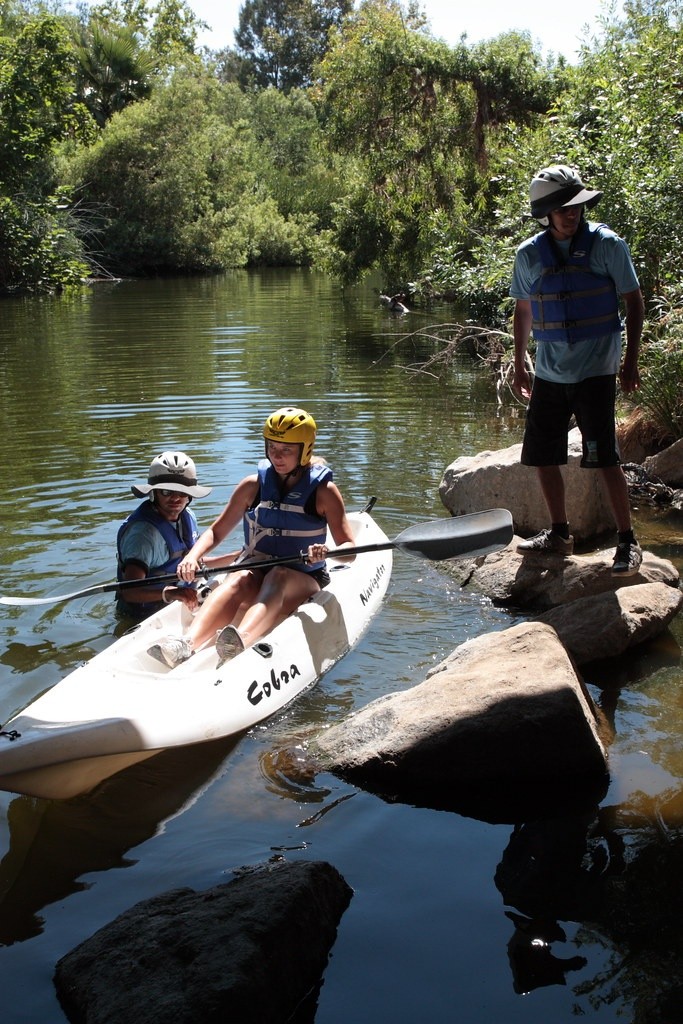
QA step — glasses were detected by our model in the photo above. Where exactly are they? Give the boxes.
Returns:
[161,489,188,499]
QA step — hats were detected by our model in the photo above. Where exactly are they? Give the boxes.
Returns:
[130,482,213,498]
[562,189,603,210]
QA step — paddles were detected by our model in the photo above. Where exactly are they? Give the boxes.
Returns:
[0,504,516,611]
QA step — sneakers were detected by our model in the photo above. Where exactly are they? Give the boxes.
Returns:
[148,638,194,670]
[215,624,251,670]
[516,527,573,556]
[611,538,643,577]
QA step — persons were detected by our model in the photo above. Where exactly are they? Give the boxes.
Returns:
[509,163,650,576]
[146,407,357,669]
[118,451,213,609]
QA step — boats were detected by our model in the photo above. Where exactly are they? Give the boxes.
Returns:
[1,494,393,801]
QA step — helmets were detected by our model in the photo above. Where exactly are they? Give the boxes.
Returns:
[147,450,197,486]
[262,407,318,466]
[530,164,587,227]
